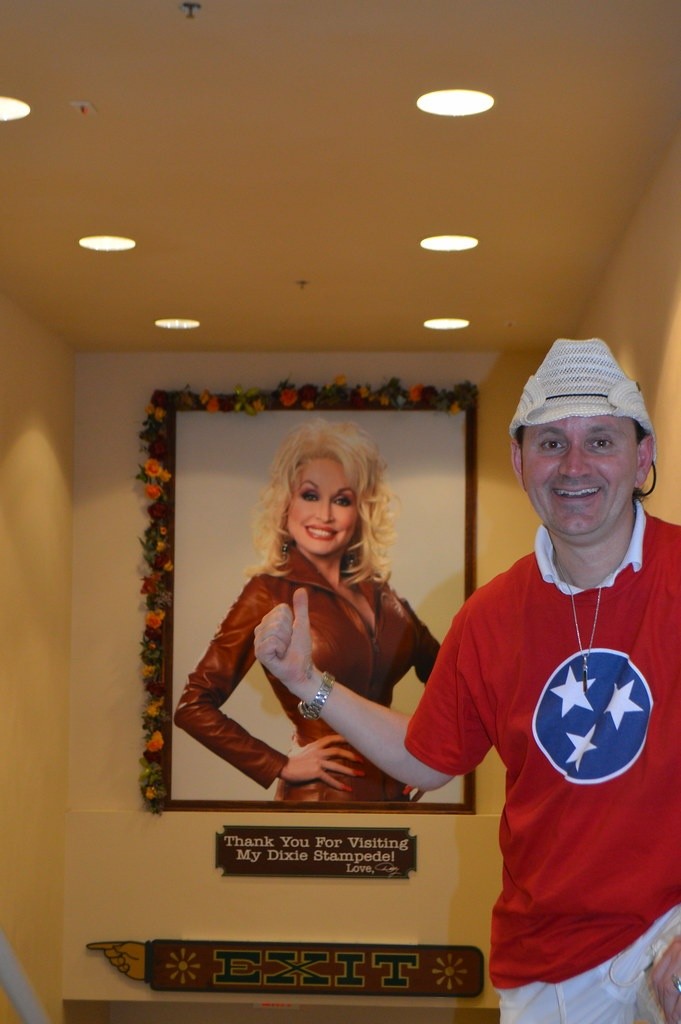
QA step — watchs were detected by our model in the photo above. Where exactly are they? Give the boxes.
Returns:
[299,672,336,720]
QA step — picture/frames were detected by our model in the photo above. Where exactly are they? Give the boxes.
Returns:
[153,390,476,814]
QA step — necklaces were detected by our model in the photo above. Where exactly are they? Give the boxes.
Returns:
[552,555,620,692]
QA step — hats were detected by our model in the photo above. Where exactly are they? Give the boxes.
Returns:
[507,337,657,466]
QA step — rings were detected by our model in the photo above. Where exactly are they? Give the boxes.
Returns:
[670,975,681,993]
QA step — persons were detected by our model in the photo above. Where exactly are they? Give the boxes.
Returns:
[165,418,448,803]
[247,337,681,1024]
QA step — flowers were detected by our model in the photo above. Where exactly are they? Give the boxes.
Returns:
[141,376,477,815]
